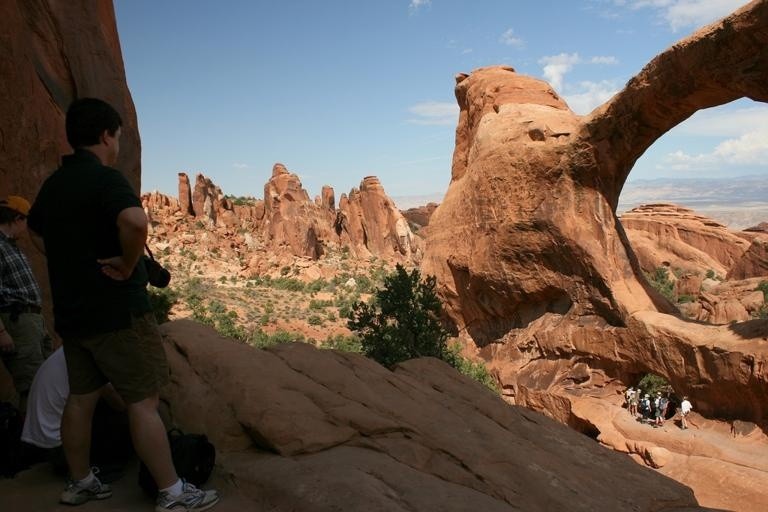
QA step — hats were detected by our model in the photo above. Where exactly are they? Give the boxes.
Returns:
[0,195,32,217]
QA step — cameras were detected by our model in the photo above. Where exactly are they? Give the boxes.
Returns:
[144,255,171,288]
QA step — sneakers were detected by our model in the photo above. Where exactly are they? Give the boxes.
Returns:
[154,477,223,512]
[58,471,113,506]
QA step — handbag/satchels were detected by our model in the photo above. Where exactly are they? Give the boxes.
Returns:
[137,426,215,501]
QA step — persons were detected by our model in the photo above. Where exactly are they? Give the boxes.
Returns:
[680,395,693,430]
[0,194,51,405]
[18,332,132,475]
[625,386,668,428]
[25,97,220,510]
